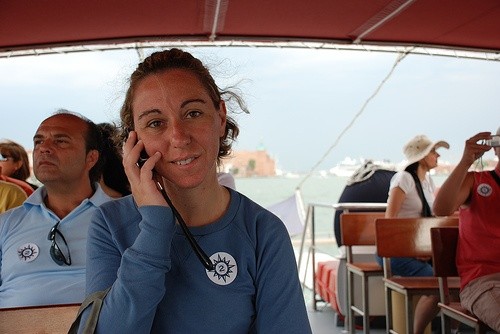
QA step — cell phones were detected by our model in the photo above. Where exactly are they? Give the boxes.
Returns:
[124,126,156,175]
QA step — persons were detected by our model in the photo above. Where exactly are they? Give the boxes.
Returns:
[76,48,312,334]
[0,140,39,212]
[0,113,115,309]
[376,134,449,334]
[433,127,500,332]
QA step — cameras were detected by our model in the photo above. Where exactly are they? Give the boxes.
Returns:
[485,135,500,146]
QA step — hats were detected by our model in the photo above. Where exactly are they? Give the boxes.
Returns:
[393,135,449,173]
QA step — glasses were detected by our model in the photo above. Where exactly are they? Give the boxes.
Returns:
[0,153,17,161]
[47,221,72,266]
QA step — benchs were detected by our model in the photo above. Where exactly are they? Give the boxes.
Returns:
[374,216,459,333]
[340,212,384,334]
[429,227,490,334]
[0,302,82,334]
